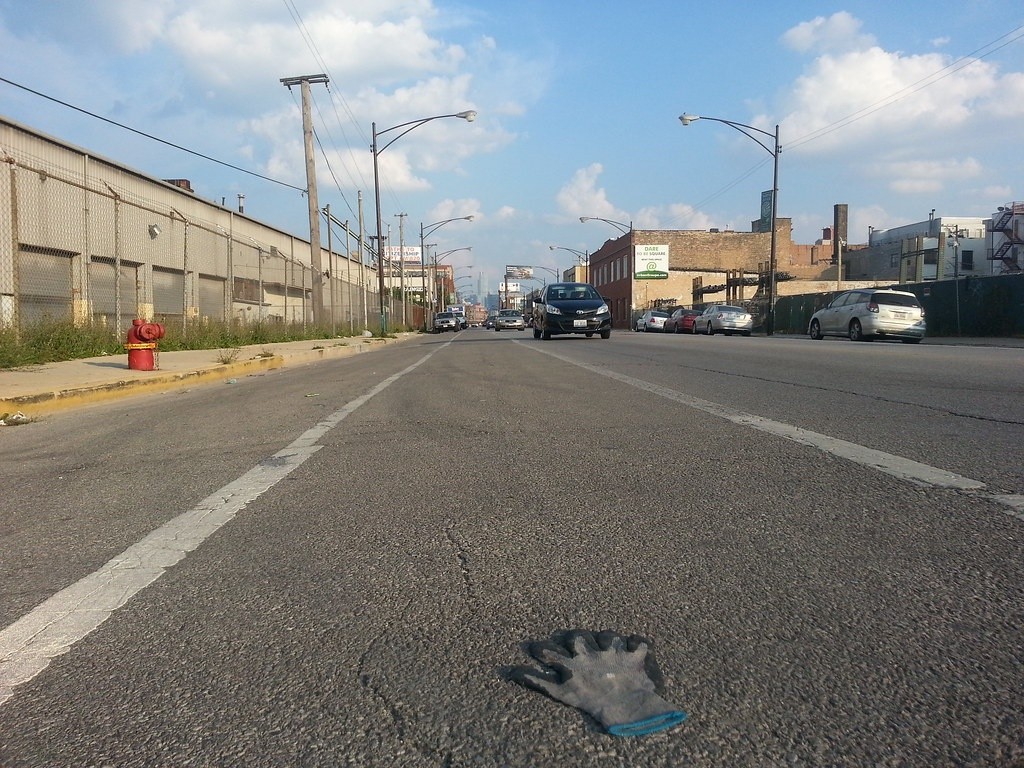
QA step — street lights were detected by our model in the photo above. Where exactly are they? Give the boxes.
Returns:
[528,265,560,282]
[433,246,473,312]
[580,216,633,333]
[678,111,780,335]
[420,215,476,335]
[370,110,478,347]
[550,246,588,282]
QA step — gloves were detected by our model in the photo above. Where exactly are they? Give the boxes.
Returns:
[509,627,688,737]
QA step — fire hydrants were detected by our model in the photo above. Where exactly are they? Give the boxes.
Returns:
[122,318,164,371]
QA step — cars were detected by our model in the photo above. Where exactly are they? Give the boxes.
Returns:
[481,309,526,332]
[452,312,467,329]
[806,288,927,345]
[663,308,704,335]
[634,310,671,332]
[530,281,613,340]
[432,312,462,333]
[691,304,754,337]
